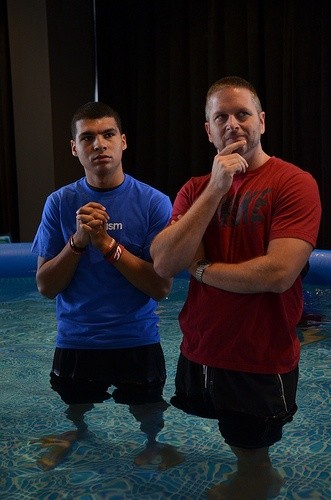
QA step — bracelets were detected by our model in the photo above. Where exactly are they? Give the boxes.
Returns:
[103,237,126,264]
[69,234,88,254]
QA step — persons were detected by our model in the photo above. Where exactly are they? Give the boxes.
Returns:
[150,75,323,499]
[30,101,174,404]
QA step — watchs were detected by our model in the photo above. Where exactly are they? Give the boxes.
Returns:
[195,258,214,284]
